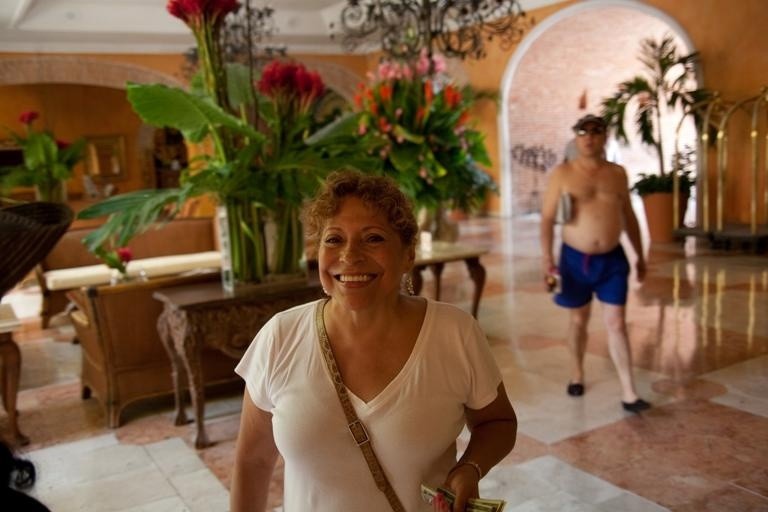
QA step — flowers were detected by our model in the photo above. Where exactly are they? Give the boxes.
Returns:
[103,248,135,277]
[253,56,331,114]
[1,112,87,204]
[352,47,475,184]
[164,0,244,44]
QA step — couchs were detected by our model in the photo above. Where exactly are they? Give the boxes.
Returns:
[64,267,243,428]
[35,217,215,334]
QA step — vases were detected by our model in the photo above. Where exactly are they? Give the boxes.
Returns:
[32,181,65,204]
[217,205,299,296]
[431,198,462,244]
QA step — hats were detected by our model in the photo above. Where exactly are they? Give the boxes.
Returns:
[570,114,606,132]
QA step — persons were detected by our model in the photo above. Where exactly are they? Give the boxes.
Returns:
[539,114,652,410]
[0,330,31,444]
[230,168,517,512]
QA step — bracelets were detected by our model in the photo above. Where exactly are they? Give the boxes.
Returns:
[457,459,485,479]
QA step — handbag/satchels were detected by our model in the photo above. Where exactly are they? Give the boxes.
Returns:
[550,193,577,225]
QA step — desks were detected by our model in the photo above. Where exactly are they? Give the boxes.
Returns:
[151,239,491,448]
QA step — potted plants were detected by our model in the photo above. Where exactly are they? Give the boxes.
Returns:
[601,30,719,245]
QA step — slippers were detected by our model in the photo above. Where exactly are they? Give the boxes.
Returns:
[567,381,585,396]
[623,397,655,411]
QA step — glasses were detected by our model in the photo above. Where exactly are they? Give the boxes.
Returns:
[577,125,606,137]
